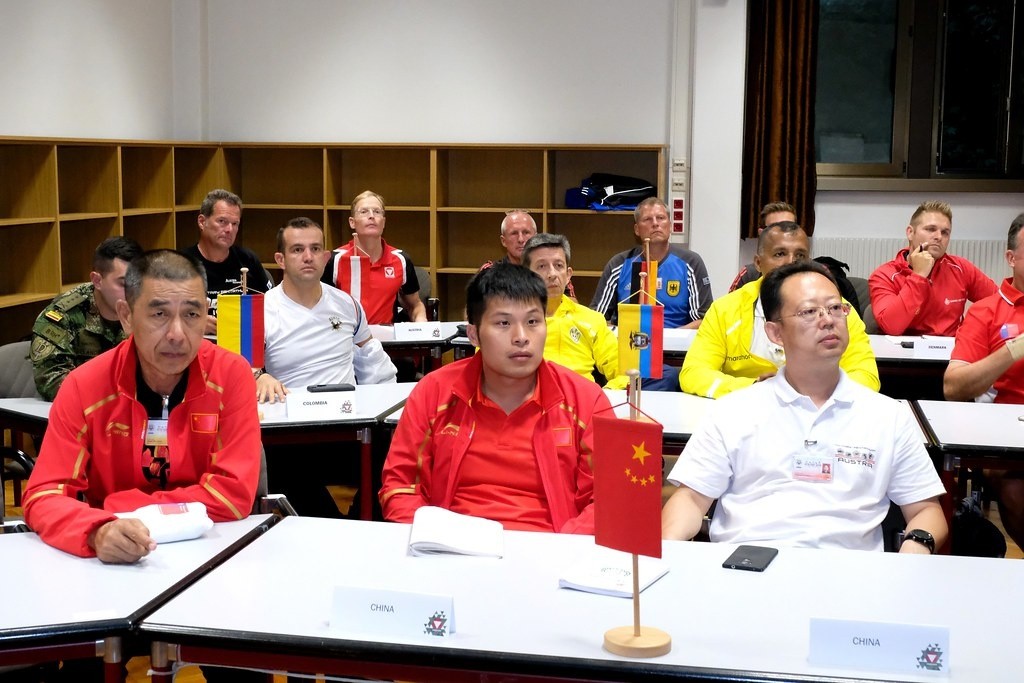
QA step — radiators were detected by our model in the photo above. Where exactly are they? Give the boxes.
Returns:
[807,238,1014,319]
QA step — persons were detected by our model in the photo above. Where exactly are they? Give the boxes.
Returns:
[587,196,713,391]
[473,232,638,390]
[728,202,798,293]
[869,198,998,403]
[176,189,272,350]
[379,262,617,536]
[249,216,398,522]
[822,464,829,473]
[943,213,1024,551]
[660,260,949,556]
[492,209,578,304]
[321,190,428,382]
[678,223,881,401]
[28,236,146,403]
[21,247,263,565]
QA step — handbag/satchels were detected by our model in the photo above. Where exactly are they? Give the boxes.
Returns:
[564,172,657,210]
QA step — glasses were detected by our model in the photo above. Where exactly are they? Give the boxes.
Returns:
[354,207,385,218]
[773,303,852,323]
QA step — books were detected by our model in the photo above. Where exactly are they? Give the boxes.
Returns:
[559,561,668,599]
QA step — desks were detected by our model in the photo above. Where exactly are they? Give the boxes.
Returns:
[0,320,1024,683]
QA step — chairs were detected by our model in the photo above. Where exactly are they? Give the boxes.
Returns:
[398,266,444,380]
[0,341,42,506]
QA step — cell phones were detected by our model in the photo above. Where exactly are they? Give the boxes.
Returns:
[307,384,355,394]
[722,546,778,572]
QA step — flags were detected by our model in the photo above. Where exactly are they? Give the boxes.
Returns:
[339,256,371,303]
[617,303,664,379]
[215,294,265,370]
[620,263,658,306]
[590,414,664,559]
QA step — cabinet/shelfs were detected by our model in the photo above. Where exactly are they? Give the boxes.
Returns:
[219,141,670,368]
[0,136,219,344]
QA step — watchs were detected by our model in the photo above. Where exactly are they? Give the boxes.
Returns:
[903,529,935,554]
